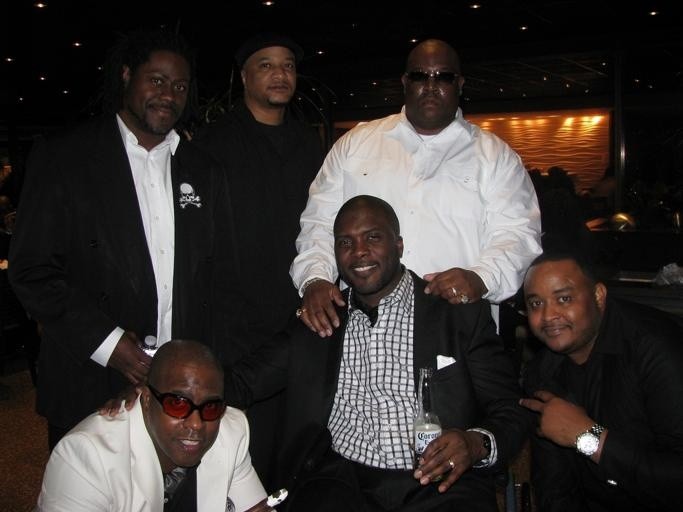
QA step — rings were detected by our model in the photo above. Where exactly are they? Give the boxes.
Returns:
[447,459,455,471]
[294,308,305,318]
[451,286,457,298]
[459,293,468,304]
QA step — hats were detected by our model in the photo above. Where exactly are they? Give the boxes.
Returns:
[233,18,302,70]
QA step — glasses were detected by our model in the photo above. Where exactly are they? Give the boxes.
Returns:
[404,71,461,85]
[144,381,227,422]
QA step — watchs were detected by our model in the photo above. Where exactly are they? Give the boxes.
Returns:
[573,423,604,459]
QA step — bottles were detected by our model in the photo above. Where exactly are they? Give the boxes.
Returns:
[413,368,442,483]
[141,334,158,356]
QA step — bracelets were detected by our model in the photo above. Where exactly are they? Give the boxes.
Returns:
[297,278,322,289]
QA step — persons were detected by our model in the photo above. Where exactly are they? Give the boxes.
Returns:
[221,193,537,512]
[517,250,681,512]
[4,42,239,456]
[198,26,326,439]
[287,36,547,341]
[32,338,282,512]
